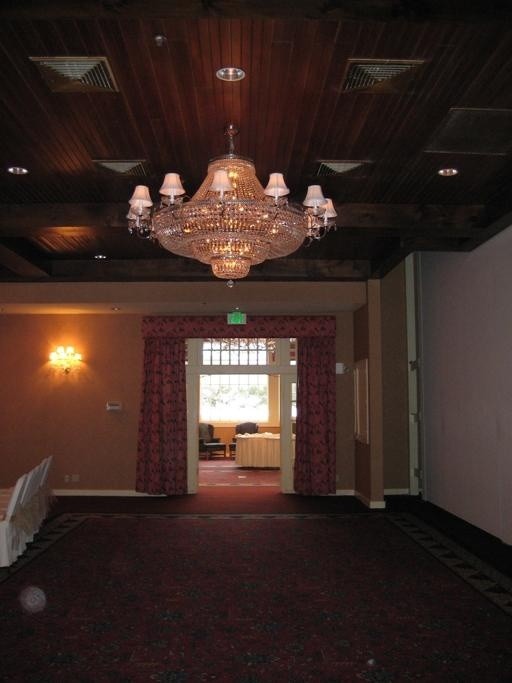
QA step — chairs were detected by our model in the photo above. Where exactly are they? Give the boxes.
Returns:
[229,421,259,461]
[198,422,226,459]
[1,451,54,567]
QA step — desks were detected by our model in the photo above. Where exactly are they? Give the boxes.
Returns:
[233,431,297,469]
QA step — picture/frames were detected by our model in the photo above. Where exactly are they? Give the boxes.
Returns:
[351,357,371,446]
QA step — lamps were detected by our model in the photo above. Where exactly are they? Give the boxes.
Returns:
[48,344,83,374]
[123,124,339,290]
